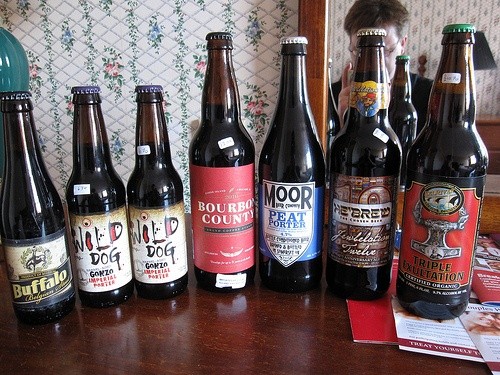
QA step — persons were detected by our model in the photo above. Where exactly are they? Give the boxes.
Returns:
[327,0,436,137]
[470,312,500,336]
[460,311,496,332]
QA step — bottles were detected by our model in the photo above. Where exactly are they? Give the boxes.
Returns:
[388,54,420,191]
[325,56,343,189]
[329,24,403,300]
[0,88,79,324]
[393,23,490,322]
[61,85,135,309]
[255,34,326,296]
[124,85,189,299]
[188,31,258,295]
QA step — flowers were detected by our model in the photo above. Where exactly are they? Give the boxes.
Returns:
[104,63,120,76]
[247,100,265,115]
[112,137,123,153]
[66,99,75,113]
[149,25,161,40]
[197,61,207,76]
[29,64,39,79]
[18,0,26,7]
[38,135,43,144]
[246,21,260,38]
[163,100,168,113]
[62,32,72,45]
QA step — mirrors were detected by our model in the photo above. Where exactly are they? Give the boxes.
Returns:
[298,0,500,177]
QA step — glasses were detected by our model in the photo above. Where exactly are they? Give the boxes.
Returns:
[349,38,399,56]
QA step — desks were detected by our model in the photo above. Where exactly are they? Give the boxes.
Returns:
[0,210,500,374]
